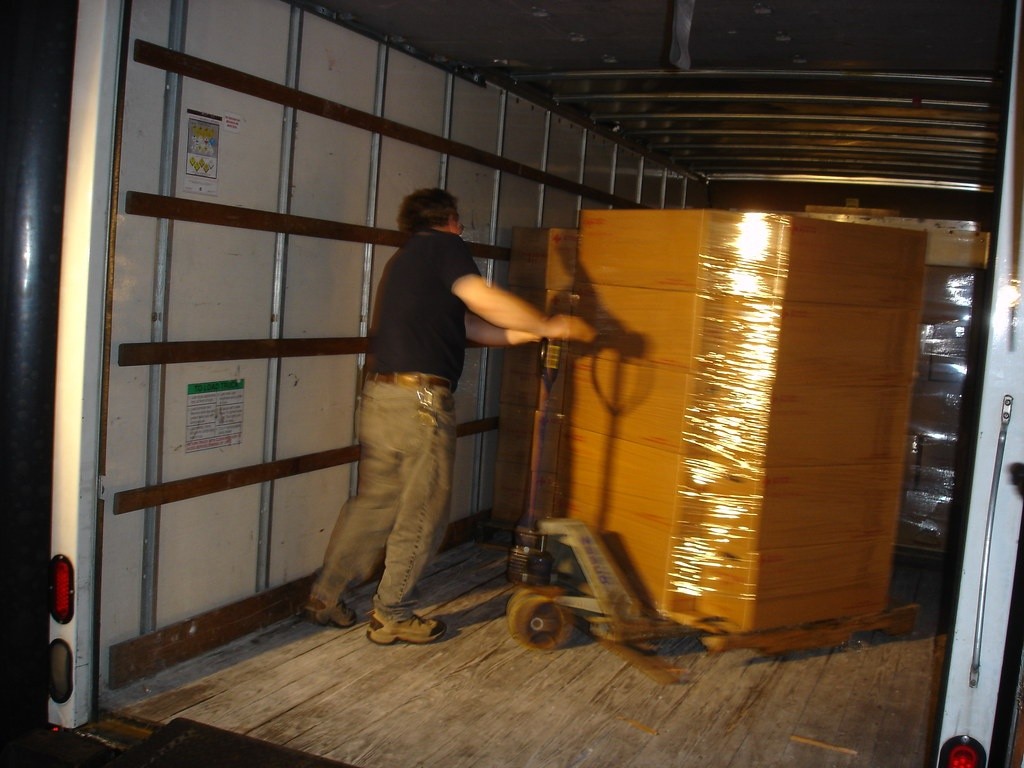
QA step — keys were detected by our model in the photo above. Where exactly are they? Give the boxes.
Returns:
[411,407,439,434]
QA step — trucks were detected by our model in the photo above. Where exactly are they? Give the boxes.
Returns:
[50,0,1024,765]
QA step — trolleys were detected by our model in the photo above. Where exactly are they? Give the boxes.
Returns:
[504,329,921,683]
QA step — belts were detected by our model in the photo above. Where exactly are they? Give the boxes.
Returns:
[366,372,452,391]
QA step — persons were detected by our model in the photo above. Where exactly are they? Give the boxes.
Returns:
[304,188,596,646]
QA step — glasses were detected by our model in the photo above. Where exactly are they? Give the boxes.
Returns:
[445,214,465,236]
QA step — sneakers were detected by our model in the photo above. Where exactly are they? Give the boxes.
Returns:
[301,592,357,628]
[366,610,448,646]
